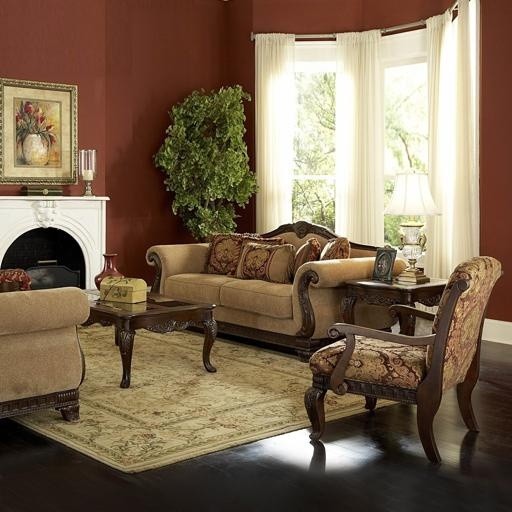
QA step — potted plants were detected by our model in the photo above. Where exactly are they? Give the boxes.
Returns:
[150,83,261,244]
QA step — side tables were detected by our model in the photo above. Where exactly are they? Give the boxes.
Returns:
[338,277,449,338]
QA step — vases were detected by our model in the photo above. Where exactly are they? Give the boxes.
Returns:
[94,253,124,292]
[20,133,49,165]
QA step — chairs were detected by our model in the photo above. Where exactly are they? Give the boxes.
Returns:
[303,255,504,465]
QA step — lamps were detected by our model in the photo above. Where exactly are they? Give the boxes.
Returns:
[382,173,440,286]
[80,148,96,197]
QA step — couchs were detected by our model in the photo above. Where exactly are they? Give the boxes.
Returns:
[0,268,91,423]
[146,221,408,365]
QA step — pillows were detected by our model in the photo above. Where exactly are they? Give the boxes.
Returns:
[204,232,261,275]
[292,238,322,283]
[319,238,351,261]
[234,242,296,284]
[234,236,287,278]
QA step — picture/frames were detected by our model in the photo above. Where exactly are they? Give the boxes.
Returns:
[372,247,397,282]
[0,78,81,186]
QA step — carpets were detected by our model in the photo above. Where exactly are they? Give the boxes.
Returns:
[6,294,402,474]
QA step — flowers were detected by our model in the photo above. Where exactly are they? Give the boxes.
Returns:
[14,99,57,154]
